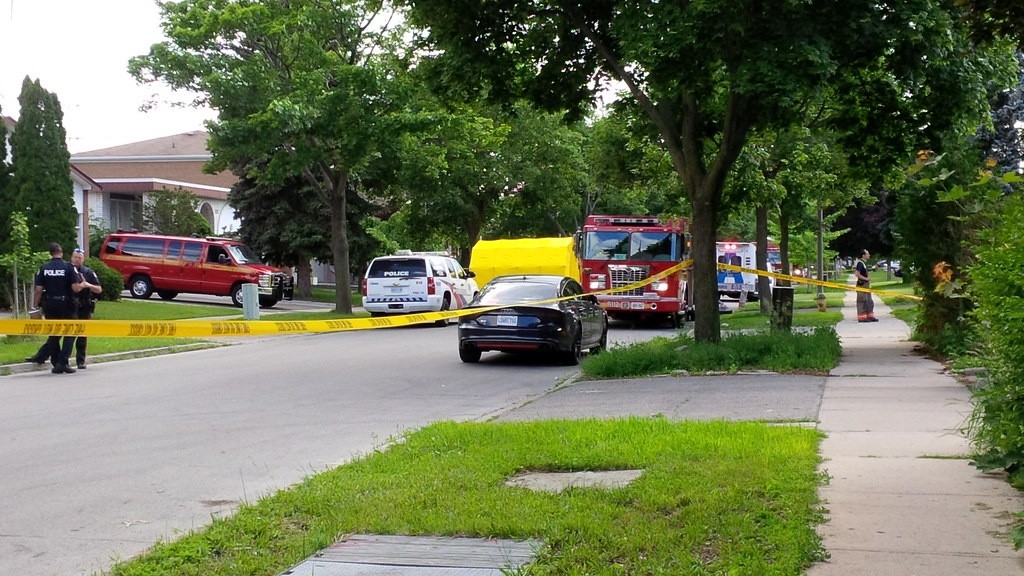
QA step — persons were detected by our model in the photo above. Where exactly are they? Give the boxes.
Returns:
[25,242,102,374]
[855,249,879,322]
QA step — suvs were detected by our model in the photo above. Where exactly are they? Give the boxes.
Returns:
[98,229,297,312]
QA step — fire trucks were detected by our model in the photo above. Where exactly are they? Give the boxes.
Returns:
[751,241,805,285]
[576,215,693,329]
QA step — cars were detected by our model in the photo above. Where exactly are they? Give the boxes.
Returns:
[877,260,905,275]
[458,274,609,367]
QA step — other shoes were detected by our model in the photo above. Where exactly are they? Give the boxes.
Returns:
[859,318,871,322]
[54,362,76,373]
[24,357,45,364]
[52,367,63,373]
[77,361,86,369]
[868,317,879,322]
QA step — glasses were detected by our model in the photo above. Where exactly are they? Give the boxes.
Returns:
[74,248,86,254]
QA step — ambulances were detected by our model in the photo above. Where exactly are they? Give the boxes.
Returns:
[716,242,776,302]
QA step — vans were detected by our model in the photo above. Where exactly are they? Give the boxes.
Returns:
[360,249,483,328]
